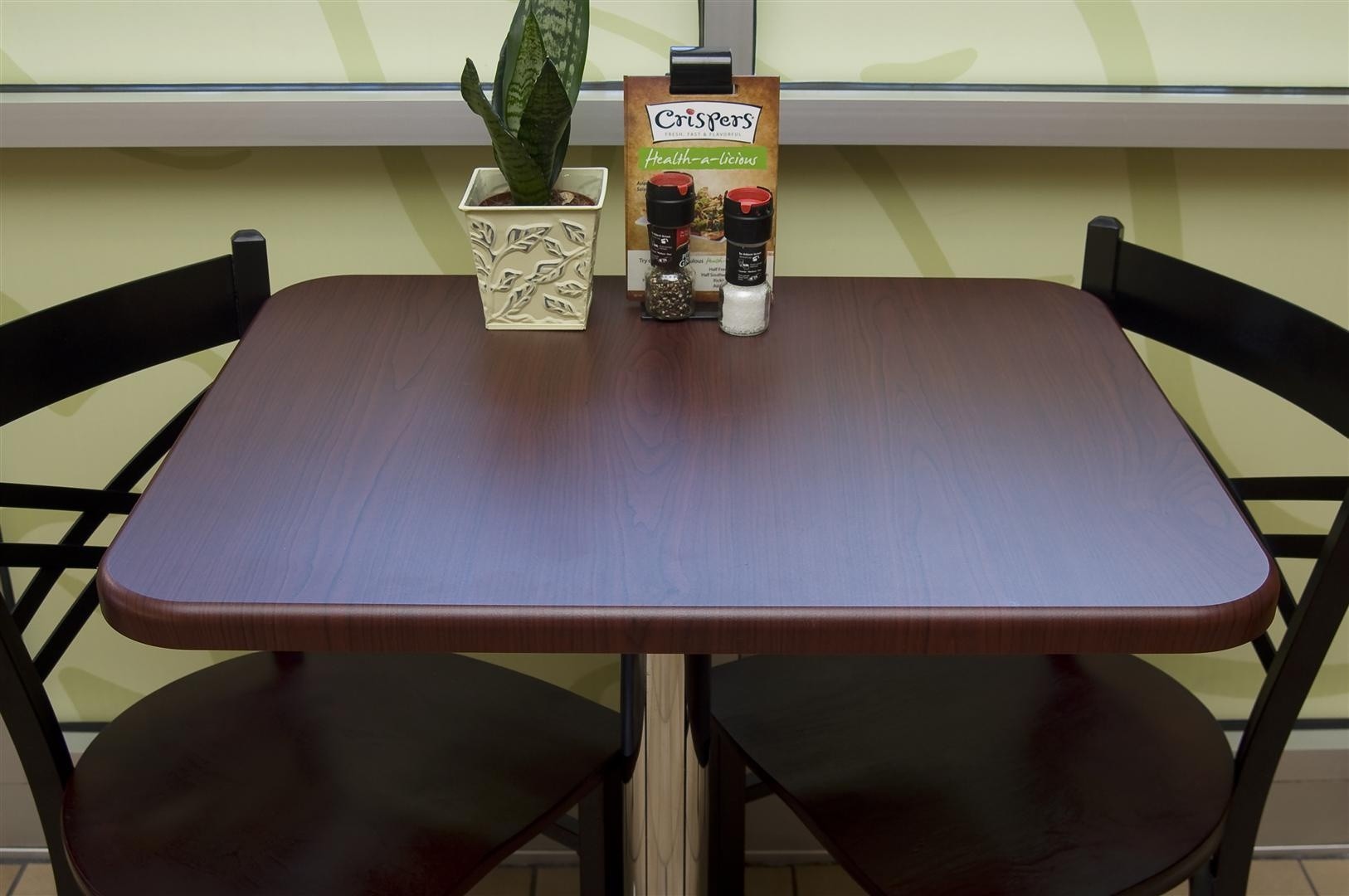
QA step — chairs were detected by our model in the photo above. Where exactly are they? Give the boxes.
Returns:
[1,229,625,895]
[709,217,1348,896]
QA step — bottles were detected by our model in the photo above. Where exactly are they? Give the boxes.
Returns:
[642,170,695,320]
[717,185,774,338]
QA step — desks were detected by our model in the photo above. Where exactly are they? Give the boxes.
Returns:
[95,274,1285,896]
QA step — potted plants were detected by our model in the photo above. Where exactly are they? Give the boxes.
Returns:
[454,0,610,331]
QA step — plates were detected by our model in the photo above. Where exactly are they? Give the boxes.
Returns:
[635,215,727,243]
